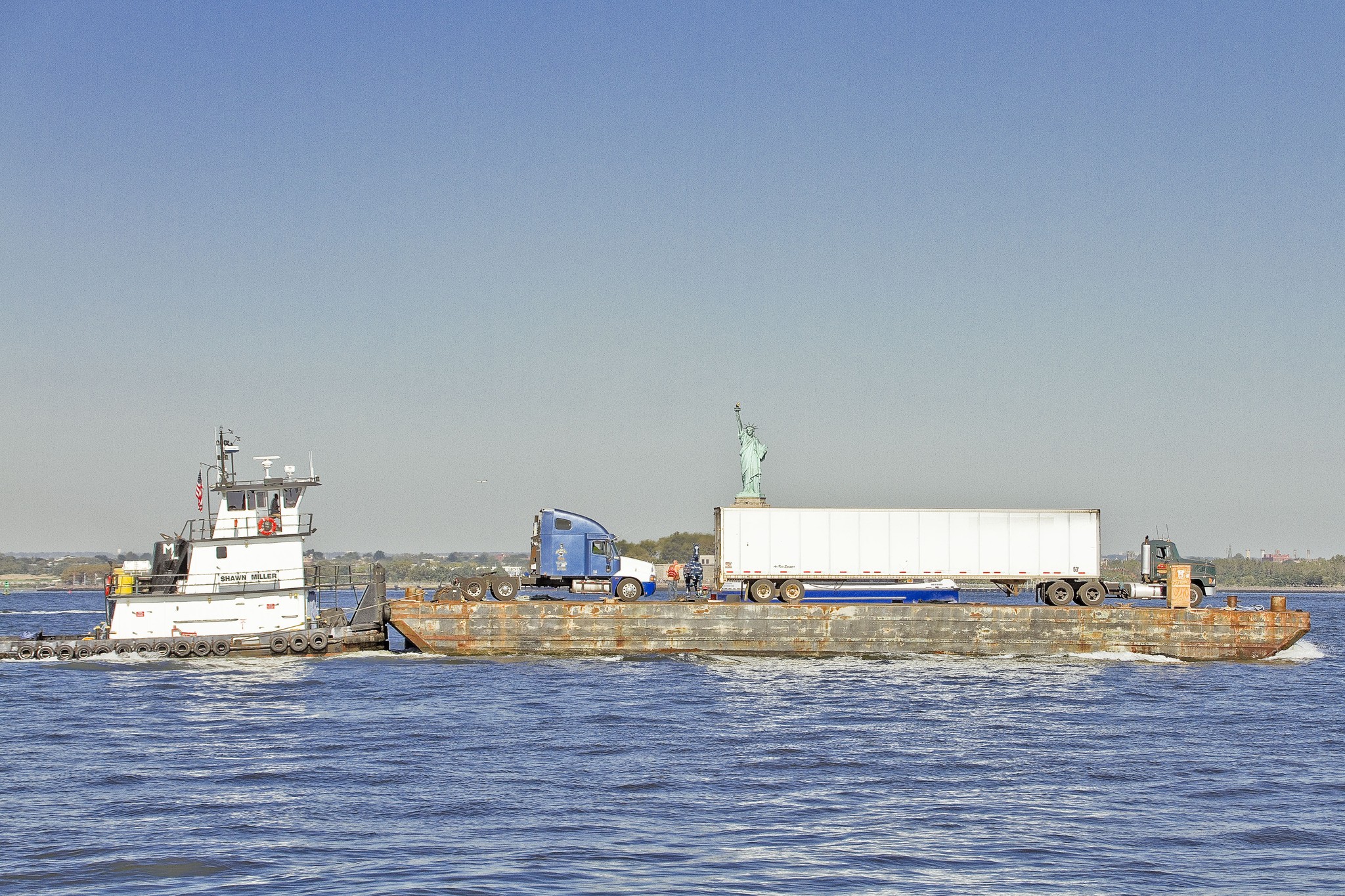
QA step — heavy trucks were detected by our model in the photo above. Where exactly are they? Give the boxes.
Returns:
[458,507,656,603]
[713,506,1217,608]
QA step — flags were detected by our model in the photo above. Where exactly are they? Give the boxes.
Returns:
[196,464,204,513]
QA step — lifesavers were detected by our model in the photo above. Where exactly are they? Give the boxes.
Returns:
[258,518,277,535]
[174,640,191,657]
[213,639,230,656]
[94,645,111,656]
[56,645,73,660]
[193,641,211,656]
[383,604,391,621]
[289,634,308,652]
[17,645,34,660]
[270,636,288,653]
[310,632,329,651]
[154,641,171,657]
[75,645,92,660]
[105,576,111,595]
[135,643,151,654]
[115,644,132,654]
[36,646,55,659]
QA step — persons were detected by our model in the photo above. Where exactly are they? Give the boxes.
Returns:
[270,494,280,519]
[666,560,685,602]
[593,542,599,550]
[734,402,768,498]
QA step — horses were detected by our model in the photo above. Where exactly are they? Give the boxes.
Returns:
[682,542,704,598]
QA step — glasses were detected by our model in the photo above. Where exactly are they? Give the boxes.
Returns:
[675,561,678,563]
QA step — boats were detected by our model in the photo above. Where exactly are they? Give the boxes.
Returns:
[0,425,392,659]
[391,595,1312,659]
